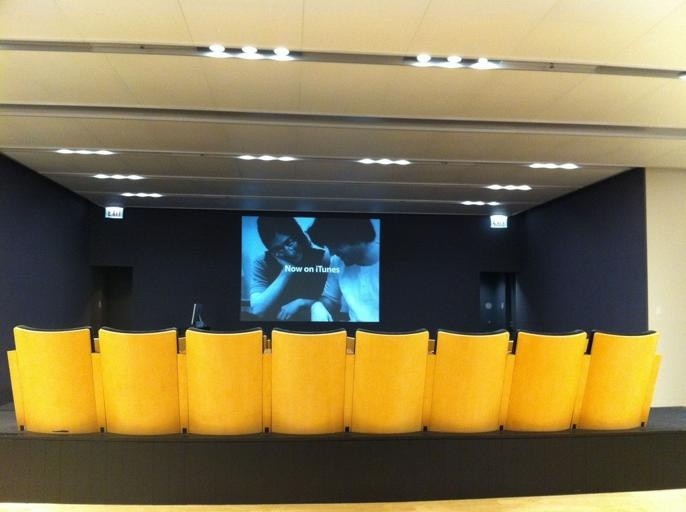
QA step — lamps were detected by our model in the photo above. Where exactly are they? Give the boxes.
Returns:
[489,208,510,230]
[104,203,125,219]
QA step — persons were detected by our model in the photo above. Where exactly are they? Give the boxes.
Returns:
[247,216,331,322]
[304,216,380,323]
